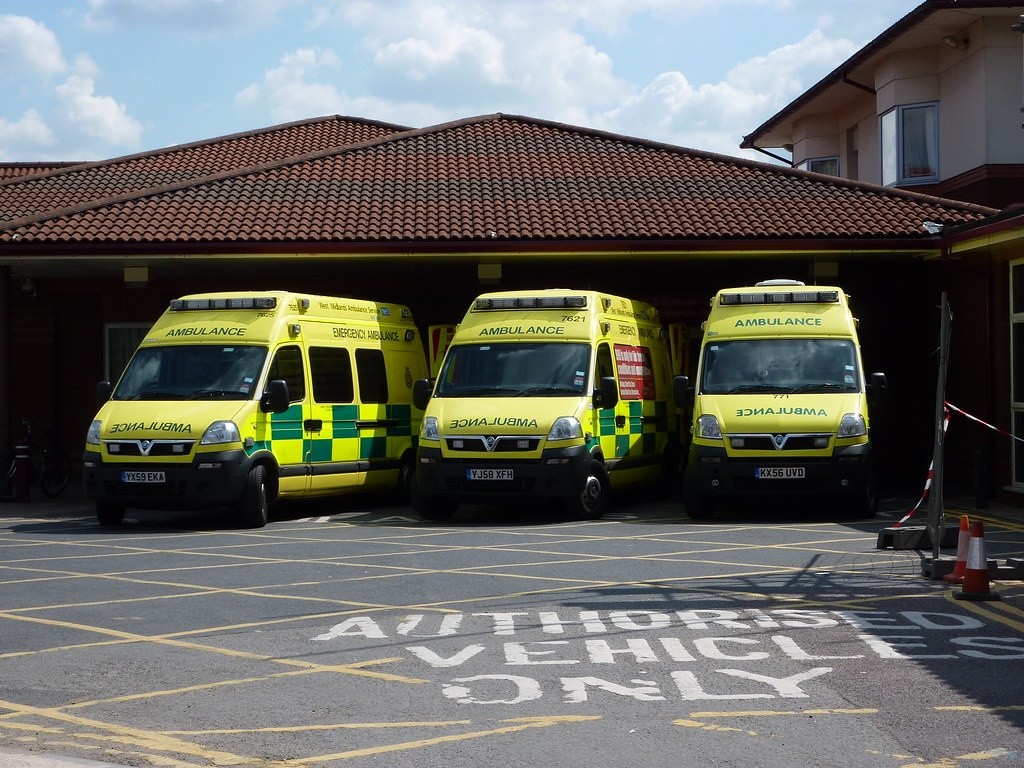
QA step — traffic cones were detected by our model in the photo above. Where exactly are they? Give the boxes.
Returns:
[951,522,1001,601]
[943,515,973,585]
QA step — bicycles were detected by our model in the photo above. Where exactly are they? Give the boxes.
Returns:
[0,420,71,505]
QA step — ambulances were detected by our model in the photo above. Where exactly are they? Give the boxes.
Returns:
[409,284,692,522]
[78,289,434,529]
[674,277,897,526]
